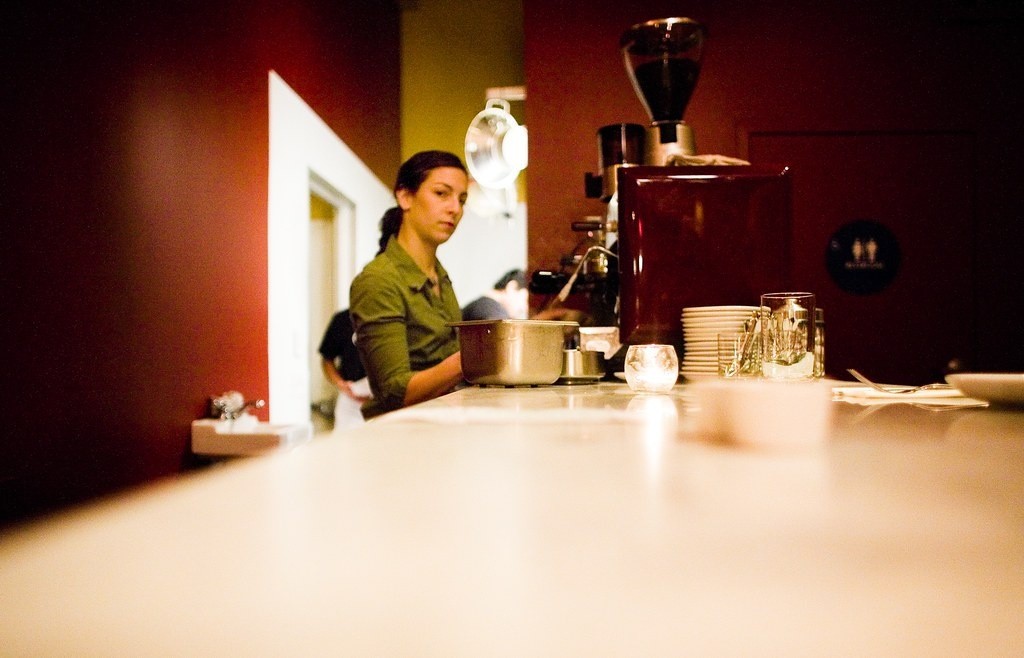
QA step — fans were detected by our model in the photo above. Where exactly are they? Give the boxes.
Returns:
[462,107,528,190]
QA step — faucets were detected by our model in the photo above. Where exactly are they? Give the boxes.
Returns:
[221,400,265,420]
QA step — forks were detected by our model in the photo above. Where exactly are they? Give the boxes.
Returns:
[846,368,956,397]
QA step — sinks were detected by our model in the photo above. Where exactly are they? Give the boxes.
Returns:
[191,419,313,455]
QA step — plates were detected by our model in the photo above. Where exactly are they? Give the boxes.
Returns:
[679,305,772,383]
[944,374,1024,406]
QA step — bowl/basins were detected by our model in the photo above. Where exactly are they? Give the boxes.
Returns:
[560,348,606,385]
[444,320,579,385]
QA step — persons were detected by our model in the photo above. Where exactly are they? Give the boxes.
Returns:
[317,309,373,435]
[350,151,586,411]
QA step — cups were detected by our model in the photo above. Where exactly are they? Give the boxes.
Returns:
[761,292,818,384]
[624,343,680,393]
[717,334,763,382]
